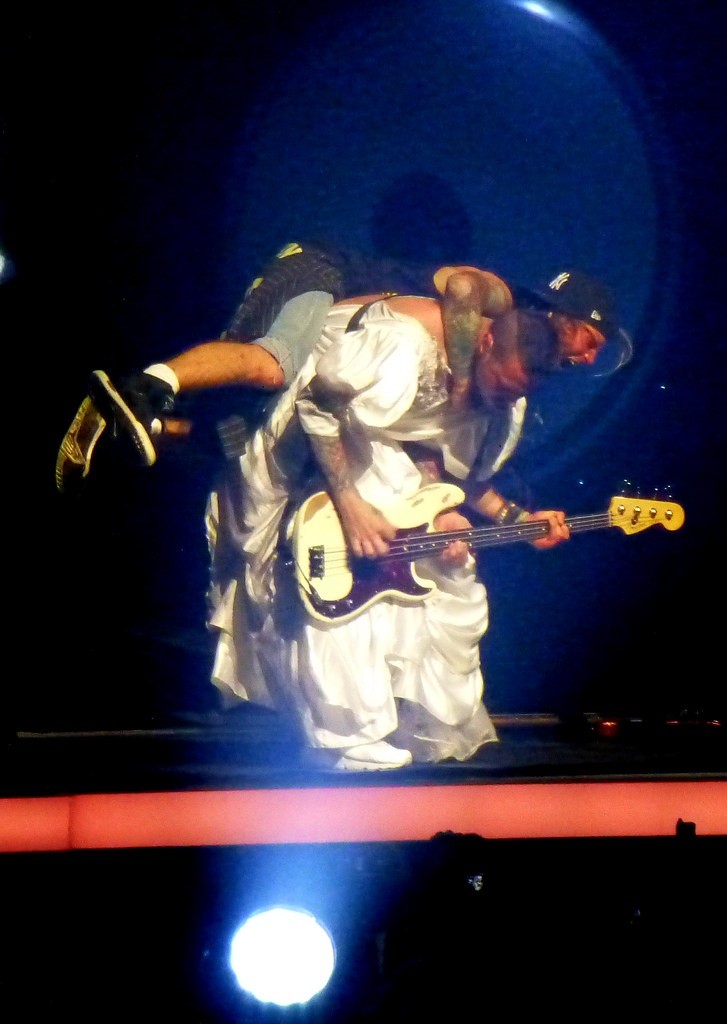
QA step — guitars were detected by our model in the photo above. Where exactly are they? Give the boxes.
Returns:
[274,476,685,627]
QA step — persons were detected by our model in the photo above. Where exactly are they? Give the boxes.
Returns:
[54,244,635,548]
[205,292,559,770]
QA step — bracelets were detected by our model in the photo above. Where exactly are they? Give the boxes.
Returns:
[495,502,529,526]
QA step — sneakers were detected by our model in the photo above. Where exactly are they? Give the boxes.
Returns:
[385,711,450,761]
[300,734,410,772]
[57,398,117,490]
[90,370,171,464]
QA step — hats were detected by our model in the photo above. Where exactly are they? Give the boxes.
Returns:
[515,269,624,338]
